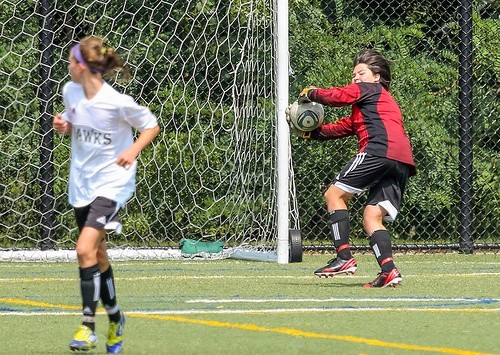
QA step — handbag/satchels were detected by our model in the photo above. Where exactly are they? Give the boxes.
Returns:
[178,238,224,254]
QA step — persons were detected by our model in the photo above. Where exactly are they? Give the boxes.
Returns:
[53,38,160,353]
[285,51,416,288]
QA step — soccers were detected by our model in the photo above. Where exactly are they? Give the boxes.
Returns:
[290,99,324,131]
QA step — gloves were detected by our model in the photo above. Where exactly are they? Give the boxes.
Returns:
[285,103,323,140]
[298,86,321,105]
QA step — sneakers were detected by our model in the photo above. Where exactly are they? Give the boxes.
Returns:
[106,310,125,354]
[314,257,357,278]
[363,267,403,289]
[70,326,97,351]
